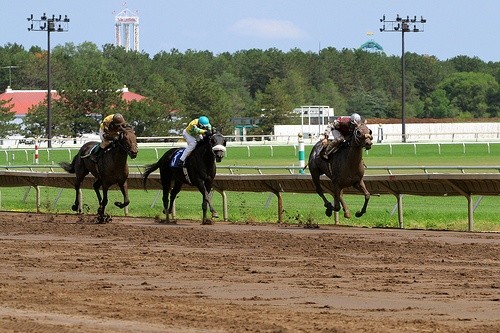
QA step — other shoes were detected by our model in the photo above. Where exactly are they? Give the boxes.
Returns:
[321,152,328,159]
[177,160,184,166]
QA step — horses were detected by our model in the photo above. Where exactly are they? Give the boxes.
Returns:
[309,120,374,218]
[59,125,138,220]
[144,127,227,224]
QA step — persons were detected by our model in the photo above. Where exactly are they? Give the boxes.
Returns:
[176,116,211,166]
[92,113,126,154]
[321,113,362,160]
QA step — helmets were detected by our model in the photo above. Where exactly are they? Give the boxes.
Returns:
[113,113,124,125]
[350,113,361,124]
[199,116,209,126]
